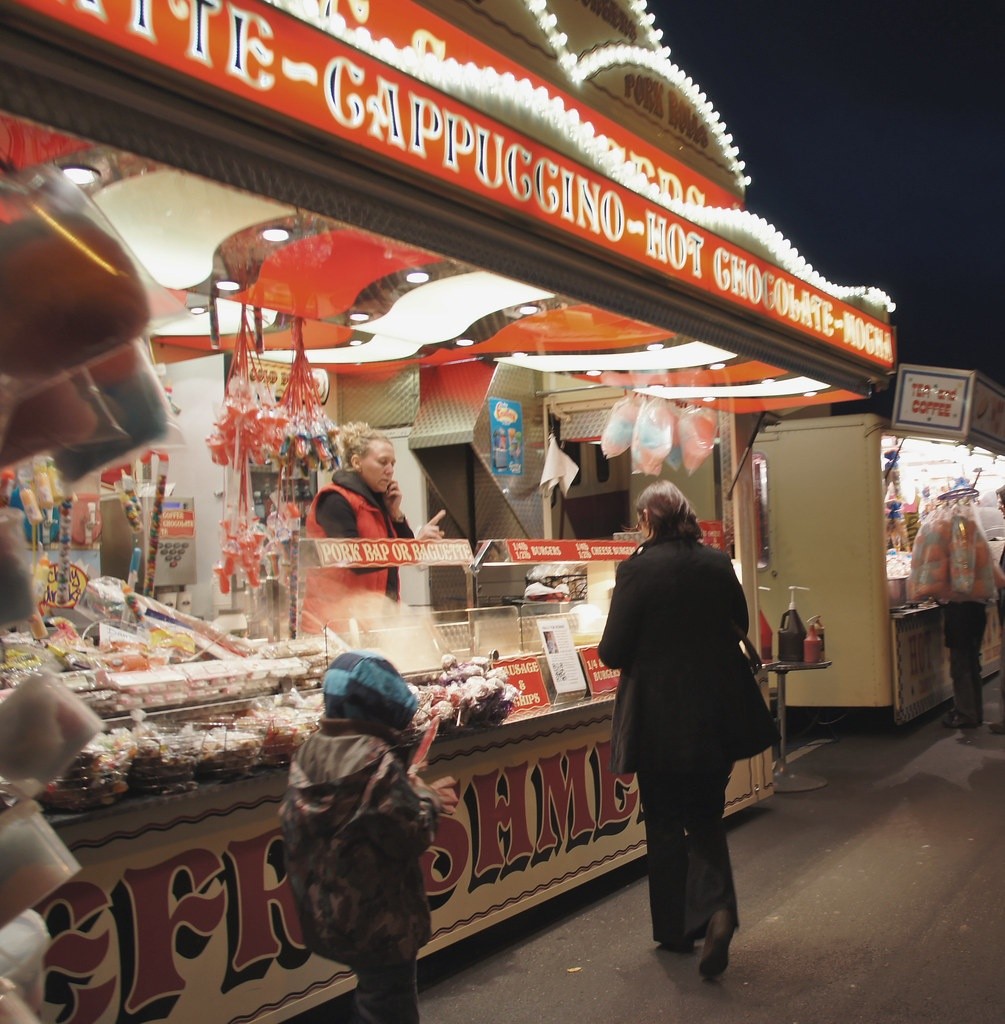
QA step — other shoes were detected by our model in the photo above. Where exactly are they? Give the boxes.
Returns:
[699,909,733,978]
[989,722,1005,734]
[941,711,983,728]
[658,939,694,952]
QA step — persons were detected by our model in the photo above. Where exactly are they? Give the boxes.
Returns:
[990,485,1005,735]
[279,651,443,1024]
[598,481,778,981]
[298,422,446,637]
[936,601,988,725]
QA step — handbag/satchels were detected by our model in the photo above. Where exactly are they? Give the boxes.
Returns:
[726,623,783,761]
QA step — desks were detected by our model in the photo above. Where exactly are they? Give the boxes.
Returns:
[766,659,832,792]
